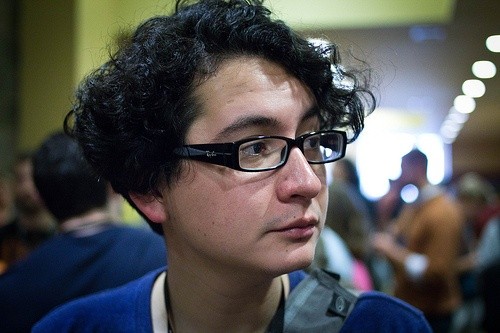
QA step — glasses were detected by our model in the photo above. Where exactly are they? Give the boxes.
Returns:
[169,130,347,172]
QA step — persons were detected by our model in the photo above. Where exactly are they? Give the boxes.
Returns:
[0,127,167,333]
[30,0,430,333]
[314,148,500,333]
